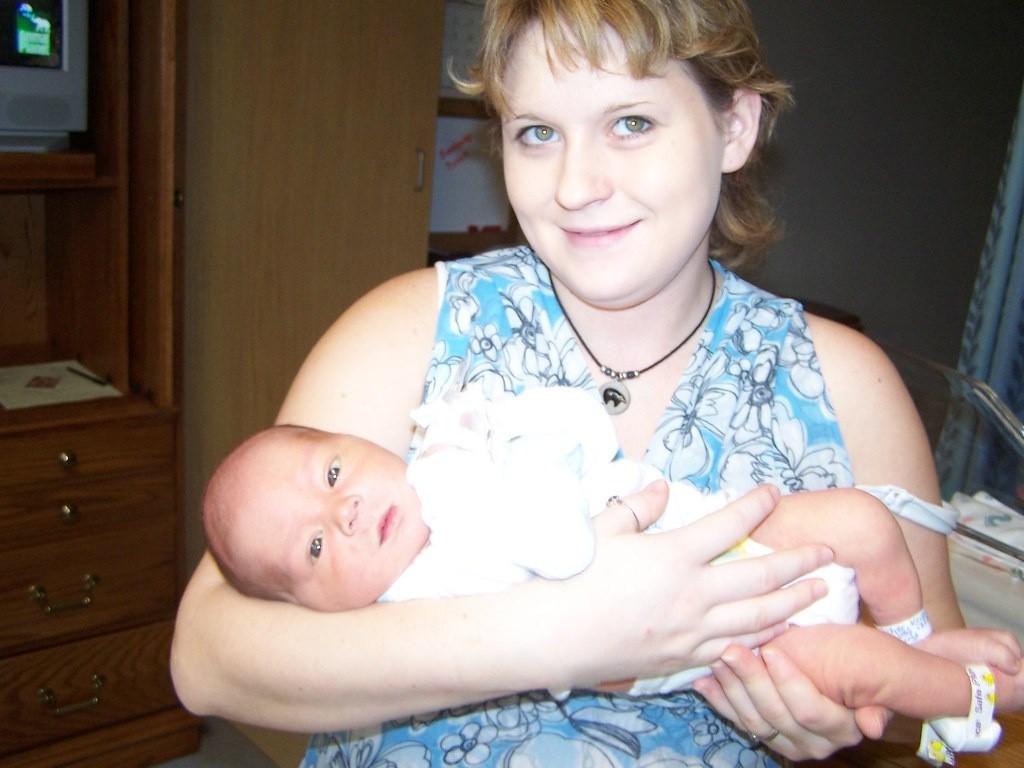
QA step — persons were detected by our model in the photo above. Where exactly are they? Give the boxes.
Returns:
[200,420,1024,720]
[172,0,970,768]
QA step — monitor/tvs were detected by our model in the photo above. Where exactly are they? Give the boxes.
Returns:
[0,0,89,152]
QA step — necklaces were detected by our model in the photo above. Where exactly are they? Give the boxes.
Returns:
[546,258,718,415]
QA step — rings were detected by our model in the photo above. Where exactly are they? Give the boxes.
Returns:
[751,730,780,746]
[606,494,640,532]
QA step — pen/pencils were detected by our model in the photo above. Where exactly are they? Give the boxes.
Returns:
[65,366,107,385]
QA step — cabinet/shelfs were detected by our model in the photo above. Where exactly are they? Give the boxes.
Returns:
[0,1,207,768]
